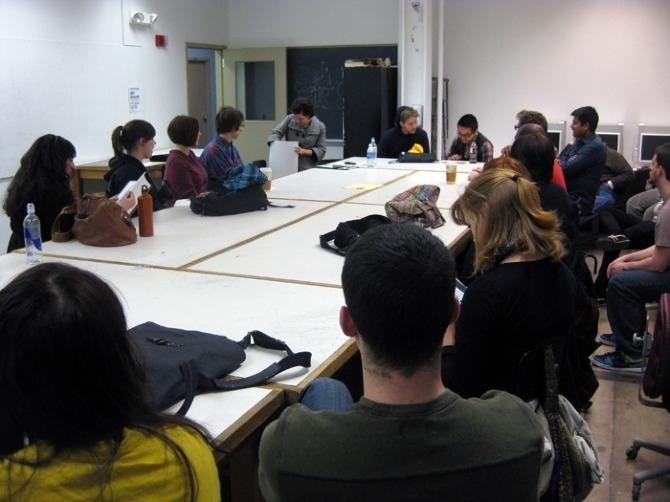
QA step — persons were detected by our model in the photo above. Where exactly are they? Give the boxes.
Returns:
[1,133,137,253]
[103,118,165,219]
[0,261,238,502]
[253,219,557,502]
[440,167,600,410]
[376,106,429,159]
[158,115,208,207]
[587,143,669,372]
[502,104,634,322]
[198,106,245,189]
[445,114,493,162]
[265,97,327,172]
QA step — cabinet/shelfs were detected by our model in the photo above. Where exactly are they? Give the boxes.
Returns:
[342,65,397,160]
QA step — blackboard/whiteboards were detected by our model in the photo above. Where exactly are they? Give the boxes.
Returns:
[245,44,399,147]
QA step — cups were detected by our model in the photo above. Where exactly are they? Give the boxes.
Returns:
[260,167,272,190]
[447,164,457,183]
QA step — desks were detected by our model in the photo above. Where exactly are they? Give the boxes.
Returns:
[262,168,415,202]
[12,195,341,271]
[178,202,471,289]
[343,171,472,209]
[78,146,204,195]
[1,252,357,460]
[318,157,485,172]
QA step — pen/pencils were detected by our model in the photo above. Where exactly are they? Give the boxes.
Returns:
[345,162,356,165]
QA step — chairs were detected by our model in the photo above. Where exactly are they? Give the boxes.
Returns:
[638,304,660,407]
[613,166,651,204]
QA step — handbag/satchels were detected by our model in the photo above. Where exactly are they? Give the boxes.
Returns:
[51,191,137,247]
[385,184,446,228]
[527,393,605,502]
[190,186,270,216]
[124,321,246,413]
[398,151,437,162]
[320,214,390,256]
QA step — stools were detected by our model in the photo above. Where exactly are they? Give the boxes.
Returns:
[579,212,599,234]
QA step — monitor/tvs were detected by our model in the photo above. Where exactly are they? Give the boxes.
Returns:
[546,130,562,155]
[638,133,670,162]
[596,133,620,153]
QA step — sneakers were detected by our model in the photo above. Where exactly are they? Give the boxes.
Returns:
[599,333,616,346]
[590,352,648,373]
[595,234,632,252]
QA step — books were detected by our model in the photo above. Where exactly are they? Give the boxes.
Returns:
[115,171,152,218]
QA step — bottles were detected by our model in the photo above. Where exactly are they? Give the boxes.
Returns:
[138,184,153,237]
[367,143,375,167]
[469,140,478,163]
[23,203,42,265]
[371,137,377,166]
